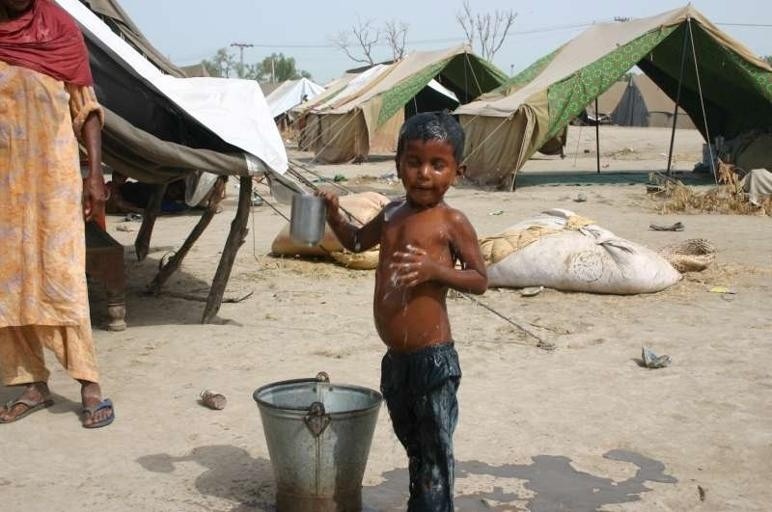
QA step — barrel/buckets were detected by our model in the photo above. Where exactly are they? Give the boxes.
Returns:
[253,370,382,512]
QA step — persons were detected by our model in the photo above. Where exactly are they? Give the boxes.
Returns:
[0,0,117,430]
[313,110,488,510]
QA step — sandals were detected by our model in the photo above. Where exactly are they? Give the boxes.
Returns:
[79,398,115,428]
[0,399,54,424]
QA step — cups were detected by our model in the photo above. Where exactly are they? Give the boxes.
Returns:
[290,192,327,246]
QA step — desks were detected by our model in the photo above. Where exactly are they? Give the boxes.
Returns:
[85,219,127,331]
